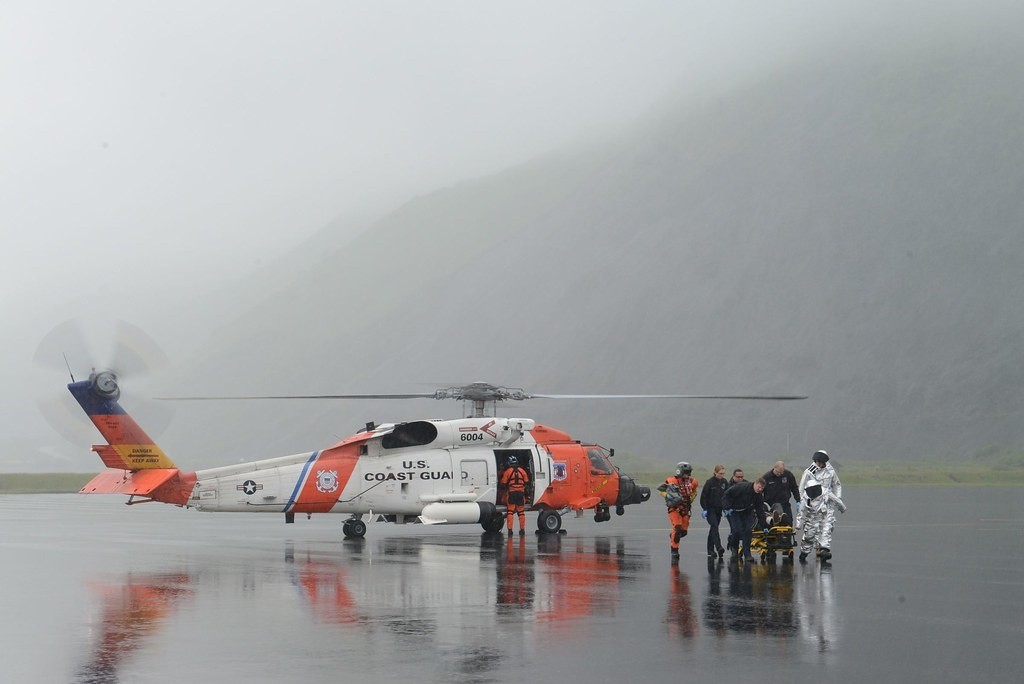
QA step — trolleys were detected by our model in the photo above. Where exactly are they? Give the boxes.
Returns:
[726,526,798,560]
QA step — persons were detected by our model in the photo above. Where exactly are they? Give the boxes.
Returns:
[750,505,787,531]
[792,480,848,560]
[762,461,800,559]
[497,459,506,504]
[721,478,769,562]
[798,449,844,556]
[656,461,699,557]
[729,469,749,487]
[501,456,529,536]
[700,464,730,557]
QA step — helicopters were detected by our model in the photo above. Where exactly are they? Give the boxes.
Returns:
[32,316,811,539]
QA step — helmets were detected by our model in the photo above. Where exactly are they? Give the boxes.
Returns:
[813,450,829,462]
[676,462,693,475]
[508,456,519,465]
[804,480,822,498]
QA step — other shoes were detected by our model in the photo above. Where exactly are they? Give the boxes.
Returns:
[708,550,717,556]
[780,513,788,525]
[671,548,679,557]
[744,553,754,561]
[519,530,525,535]
[799,552,807,559]
[508,530,513,534]
[718,547,725,557]
[820,551,831,560]
[774,511,779,523]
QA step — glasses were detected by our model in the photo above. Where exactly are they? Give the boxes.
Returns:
[735,476,743,479]
[684,470,691,474]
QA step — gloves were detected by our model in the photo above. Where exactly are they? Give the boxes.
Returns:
[702,510,708,518]
